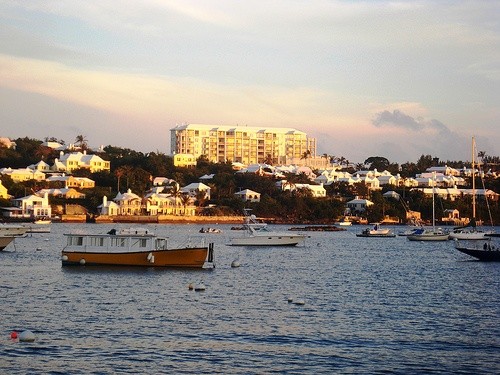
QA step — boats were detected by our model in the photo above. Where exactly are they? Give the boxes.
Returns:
[0,225,28,251]
[34,215,52,225]
[59,227,206,268]
[26,225,51,233]
[243,224,267,229]
[198,228,223,234]
[455,234,500,261]
[225,216,309,247]
[355,222,396,238]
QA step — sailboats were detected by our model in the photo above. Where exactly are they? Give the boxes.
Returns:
[399,136,497,242]
